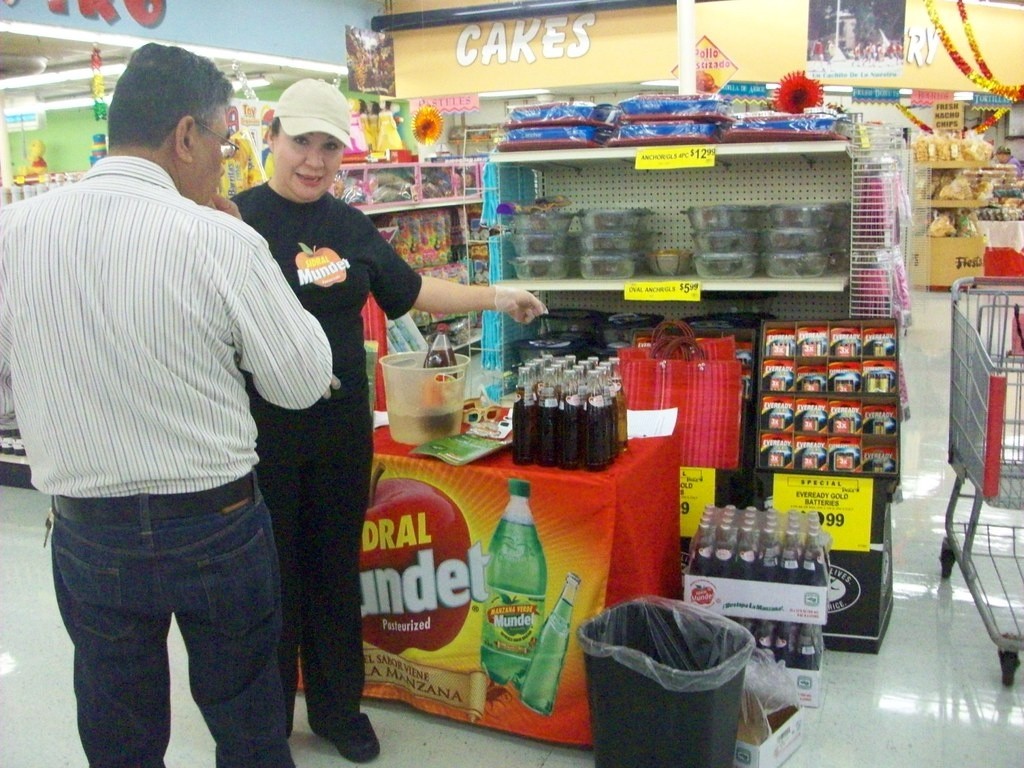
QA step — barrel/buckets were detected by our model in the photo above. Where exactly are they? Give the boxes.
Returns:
[381,351,469,446]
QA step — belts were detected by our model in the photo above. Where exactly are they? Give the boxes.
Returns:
[54,471,253,525]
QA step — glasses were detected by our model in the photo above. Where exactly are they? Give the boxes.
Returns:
[193,120,238,159]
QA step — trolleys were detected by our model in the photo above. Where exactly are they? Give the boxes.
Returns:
[939,274,1024,686]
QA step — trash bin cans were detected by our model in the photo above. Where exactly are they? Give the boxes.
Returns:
[571,594,756,768]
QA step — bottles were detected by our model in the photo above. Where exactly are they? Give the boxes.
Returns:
[688,502,831,671]
[517,570,582,718]
[419,321,454,434]
[510,353,628,473]
[482,477,547,691]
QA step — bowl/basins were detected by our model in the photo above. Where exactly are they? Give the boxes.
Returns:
[503,203,850,365]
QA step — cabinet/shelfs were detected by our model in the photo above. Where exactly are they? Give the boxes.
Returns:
[912,129,995,289]
[325,145,487,413]
[477,93,922,496]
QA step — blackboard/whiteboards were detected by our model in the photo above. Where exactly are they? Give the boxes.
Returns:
[633,144,716,170]
[770,475,873,553]
[624,281,702,302]
[680,468,715,537]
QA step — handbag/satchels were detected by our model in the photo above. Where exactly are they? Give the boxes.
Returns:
[627,337,742,469]
[620,322,739,380]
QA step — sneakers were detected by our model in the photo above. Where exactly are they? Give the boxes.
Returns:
[308,707,382,762]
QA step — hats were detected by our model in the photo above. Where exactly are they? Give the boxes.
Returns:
[274,76,354,150]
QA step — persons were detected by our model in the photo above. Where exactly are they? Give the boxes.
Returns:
[217,76,552,768]
[0,38,333,768]
[811,36,903,72]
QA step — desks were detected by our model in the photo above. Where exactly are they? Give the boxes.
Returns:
[296,414,683,750]
